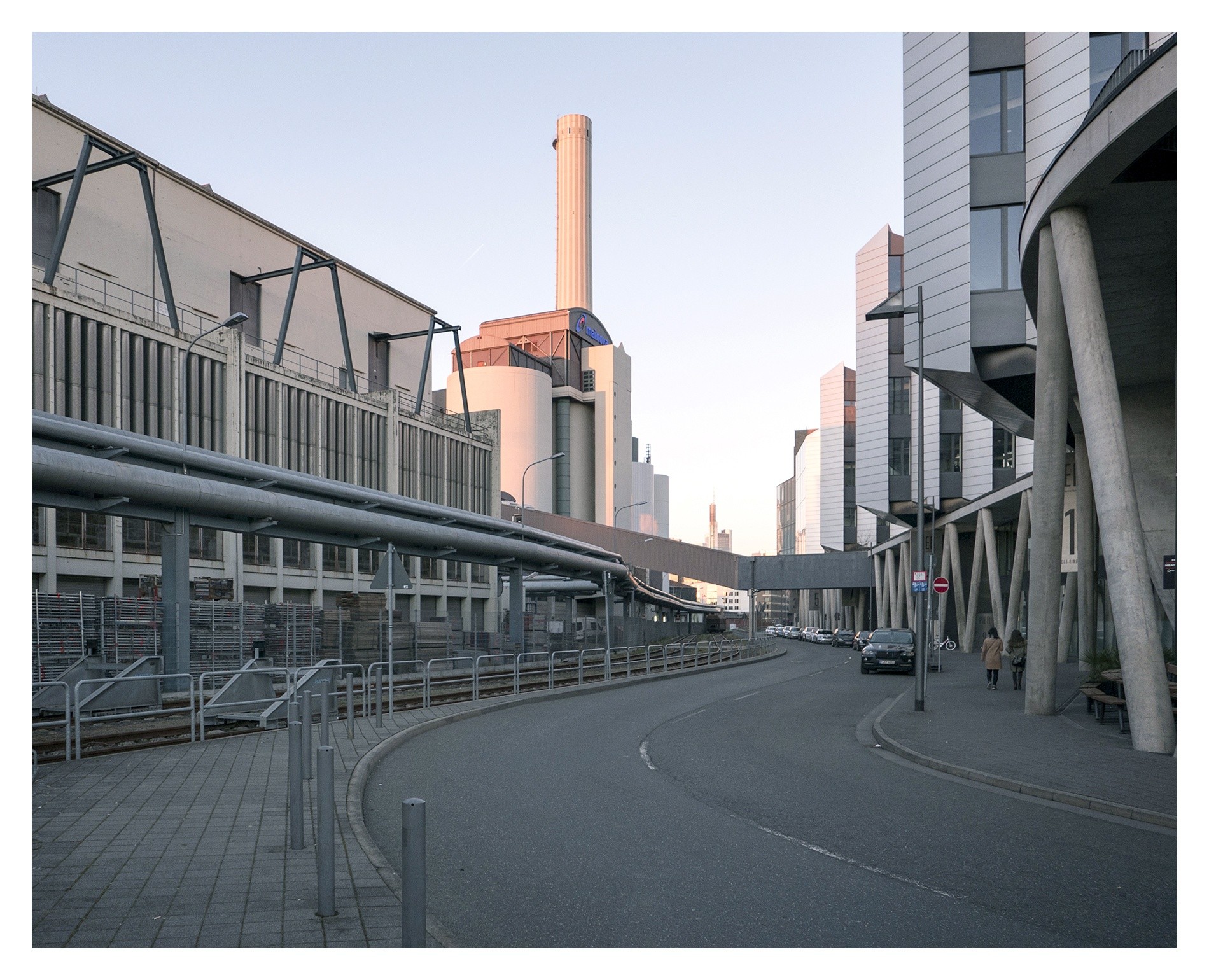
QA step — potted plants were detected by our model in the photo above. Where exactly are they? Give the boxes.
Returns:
[1076,645,1120,713]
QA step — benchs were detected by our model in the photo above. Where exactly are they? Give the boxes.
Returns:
[1079,683,1126,725]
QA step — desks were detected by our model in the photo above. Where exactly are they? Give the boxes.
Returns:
[1101,669,1131,734]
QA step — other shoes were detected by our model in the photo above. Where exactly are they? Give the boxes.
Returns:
[1014,683,1017,690]
[992,685,997,690]
[987,682,992,689]
[1018,681,1021,690]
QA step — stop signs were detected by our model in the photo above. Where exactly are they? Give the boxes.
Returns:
[933,577,949,594]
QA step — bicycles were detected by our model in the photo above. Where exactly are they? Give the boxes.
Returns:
[929,633,957,650]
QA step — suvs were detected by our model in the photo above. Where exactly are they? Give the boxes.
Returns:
[860,626,916,676]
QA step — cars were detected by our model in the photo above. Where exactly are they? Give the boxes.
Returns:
[831,628,856,648]
[852,630,872,651]
[765,624,819,643]
[813,628,834,645]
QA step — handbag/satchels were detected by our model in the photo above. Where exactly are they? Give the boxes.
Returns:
[1012,656,1025,667]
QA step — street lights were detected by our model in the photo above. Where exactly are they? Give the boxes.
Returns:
[521,452,565,527]
[180,312,247,448]
[630,538,654,566]
[613,500,648,553]
[865,284,925,711]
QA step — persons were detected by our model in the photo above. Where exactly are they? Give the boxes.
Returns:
[1006,630,1027,690]
[981,627,1003,690]
